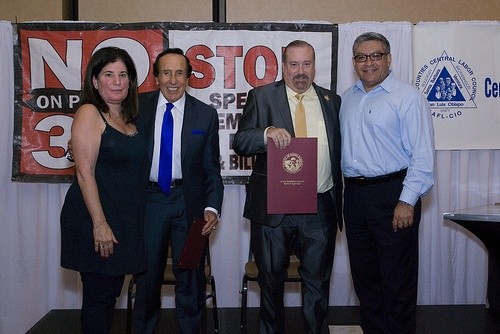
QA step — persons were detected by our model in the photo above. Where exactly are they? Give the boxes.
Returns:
[233,40,342,334]
[60,46,139,334]
[130,49,225,334]
[339,32,437,334]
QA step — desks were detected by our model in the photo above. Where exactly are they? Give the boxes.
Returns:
[443,203,500,314]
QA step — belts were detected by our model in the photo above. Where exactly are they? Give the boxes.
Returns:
[343,168,406,184]
[149,181,183,187]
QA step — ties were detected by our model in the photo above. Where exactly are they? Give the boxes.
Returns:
[294,92,307,138]
[157,103,175,194]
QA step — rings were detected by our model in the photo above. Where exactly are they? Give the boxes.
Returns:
[213,226,215,230]
[100,246,103,248]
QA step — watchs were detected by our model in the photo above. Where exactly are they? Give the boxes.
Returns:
[67,154,75,162]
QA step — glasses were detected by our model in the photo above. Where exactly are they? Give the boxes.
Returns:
[354,52,389,63]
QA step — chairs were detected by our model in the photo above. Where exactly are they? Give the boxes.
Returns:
[241,237,301,334]
[127,239,219,334]
